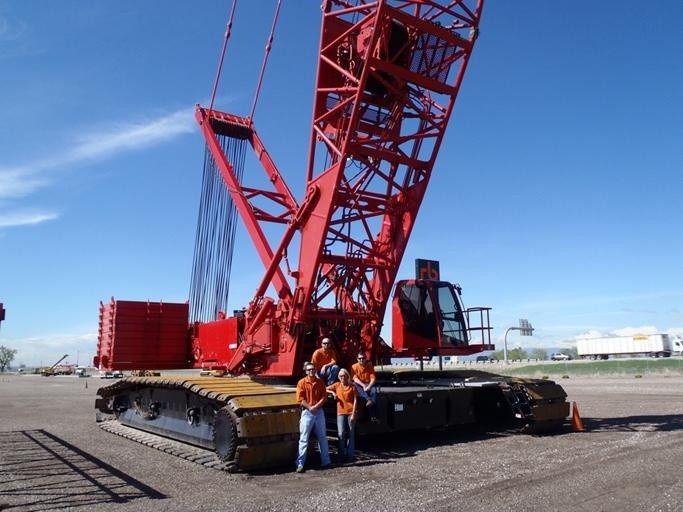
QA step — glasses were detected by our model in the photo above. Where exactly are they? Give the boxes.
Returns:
[357,356,365,360]
[322,342,331,345]
[306,368,315,371]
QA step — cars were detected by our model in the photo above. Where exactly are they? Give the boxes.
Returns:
[476,356,497,363]
[551,351,572,361]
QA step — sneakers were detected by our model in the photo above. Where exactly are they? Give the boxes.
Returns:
[365,398,376,408]
[297,466,306,473]
[322,463,337,469]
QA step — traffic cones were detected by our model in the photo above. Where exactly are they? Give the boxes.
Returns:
[570,401,585,433]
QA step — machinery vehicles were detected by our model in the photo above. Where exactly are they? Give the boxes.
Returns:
[36,353,72,377]
[94,0,569,472]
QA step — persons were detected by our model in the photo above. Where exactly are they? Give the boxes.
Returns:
[349,351,378,422]
[309,337,340,387]
[293,362,337,473]
[324,367,359,462]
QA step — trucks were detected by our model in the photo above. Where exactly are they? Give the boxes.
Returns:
[76,366,92,378]
[576,333,683,360]
